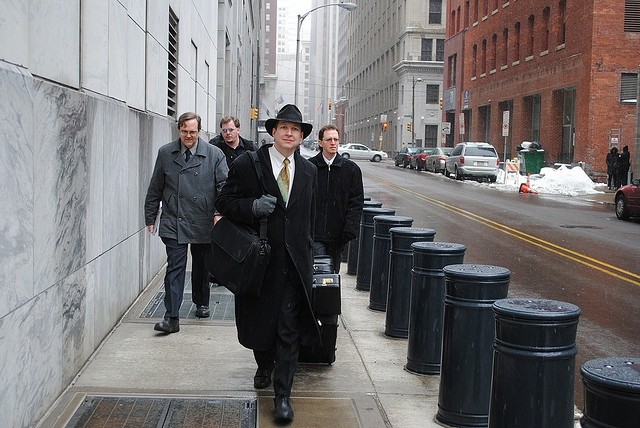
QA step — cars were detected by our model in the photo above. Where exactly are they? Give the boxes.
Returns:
[410,148,434,170]
[614,178,640,224]
[426,147,453,173]
[338,144,387,162]
[395,147,419,168]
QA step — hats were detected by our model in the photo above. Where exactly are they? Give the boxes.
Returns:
[623,145,628,151]
[265,104,313,141]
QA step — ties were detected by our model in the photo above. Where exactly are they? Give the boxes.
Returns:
[276,159,290,206]
[185,150,191,163]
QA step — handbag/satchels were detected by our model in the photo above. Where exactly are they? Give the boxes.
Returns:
[201,151,272,299]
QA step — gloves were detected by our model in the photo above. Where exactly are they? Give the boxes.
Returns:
[252,194,277,218]
[341,232,356,242]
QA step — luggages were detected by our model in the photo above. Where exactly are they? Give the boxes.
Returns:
[312,273,341,315]
[296,314,341,366]
[313,255,340,274]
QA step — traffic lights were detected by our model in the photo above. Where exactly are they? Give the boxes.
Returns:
[440,98,442,110]
[251,109,258,120]
[383,124,387,133]
[329,100,332,110]
[407,123,411,132]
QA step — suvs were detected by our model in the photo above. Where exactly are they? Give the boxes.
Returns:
[445,142,500,181]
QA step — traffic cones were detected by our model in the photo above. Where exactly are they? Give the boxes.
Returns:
[526,173,530,188]
[519,183,539,194]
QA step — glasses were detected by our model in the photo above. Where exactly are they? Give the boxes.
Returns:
[178,129,196,136]
[221,128,234,133]
[324,137,340,142]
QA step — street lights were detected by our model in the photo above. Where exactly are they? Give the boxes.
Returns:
[295,3,357,106]
[412,78,422,146]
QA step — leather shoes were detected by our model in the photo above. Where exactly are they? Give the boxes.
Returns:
[195,304,210,317]
[154,317,179,334]
[253,360,274,389]
[272,394,293,422]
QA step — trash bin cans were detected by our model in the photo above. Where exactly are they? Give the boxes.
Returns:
[520,149,545,175]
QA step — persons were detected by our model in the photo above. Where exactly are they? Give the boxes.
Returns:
[208,113,259,302]
[143,113,230,333]
[212,105,321,422]
[618,145,632,190]
[606,145,618,191]
[306,123,365,273]
